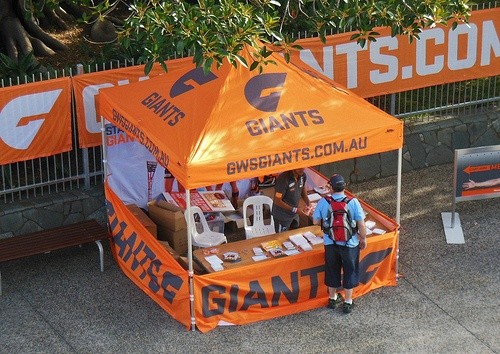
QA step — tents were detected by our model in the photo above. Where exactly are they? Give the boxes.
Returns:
[100,35,404,331]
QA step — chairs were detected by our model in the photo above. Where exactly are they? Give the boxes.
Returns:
[184,206,227,249]
[243,195,276,240]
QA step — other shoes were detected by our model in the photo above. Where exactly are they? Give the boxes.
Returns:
[327,293,344,309]
[342,301,355,313]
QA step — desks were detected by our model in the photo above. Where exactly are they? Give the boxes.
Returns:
[297,189,392,239]
[193,225,325,274]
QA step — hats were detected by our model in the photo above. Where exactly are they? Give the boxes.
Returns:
[327,174,344,186]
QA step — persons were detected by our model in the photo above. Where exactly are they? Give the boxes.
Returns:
[312,174,366,314]
[272,168,313,233]
[164,168,239,210]
[462,178,500,190]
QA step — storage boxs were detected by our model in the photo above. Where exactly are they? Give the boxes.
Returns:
[147,198,188,256]
[125,203,157,239]
[194,210,226,233]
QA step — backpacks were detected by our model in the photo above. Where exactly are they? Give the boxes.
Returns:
[321,196,358,248]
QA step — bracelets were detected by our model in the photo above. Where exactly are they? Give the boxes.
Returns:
[291,207,297,214]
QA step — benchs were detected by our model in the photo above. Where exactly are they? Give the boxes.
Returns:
[0,218,108,273]
